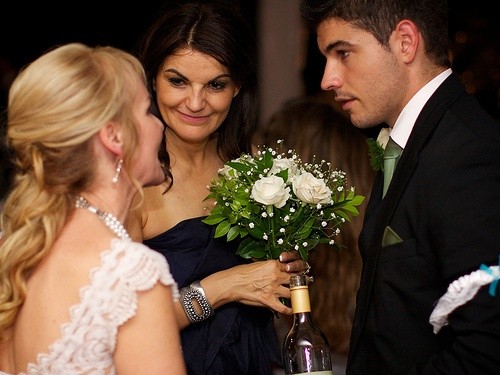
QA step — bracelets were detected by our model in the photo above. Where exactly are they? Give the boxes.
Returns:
[175,280,215,327]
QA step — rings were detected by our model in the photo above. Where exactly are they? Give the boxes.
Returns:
[308,276,315,283]
[304,262,312,275]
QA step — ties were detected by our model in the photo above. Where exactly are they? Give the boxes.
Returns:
[382,137,402,200]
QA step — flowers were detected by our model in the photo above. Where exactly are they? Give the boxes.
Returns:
[202,139,366,320]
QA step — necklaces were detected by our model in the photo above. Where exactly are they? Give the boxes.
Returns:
[72,193,133,243]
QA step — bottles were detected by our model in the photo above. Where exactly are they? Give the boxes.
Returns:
[283,275,333,375]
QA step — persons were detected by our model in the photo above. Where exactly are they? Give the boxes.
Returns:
[242,94,374,375]
[302,0,500,375]
[122,3,315,375]
[1,43,192,375]
